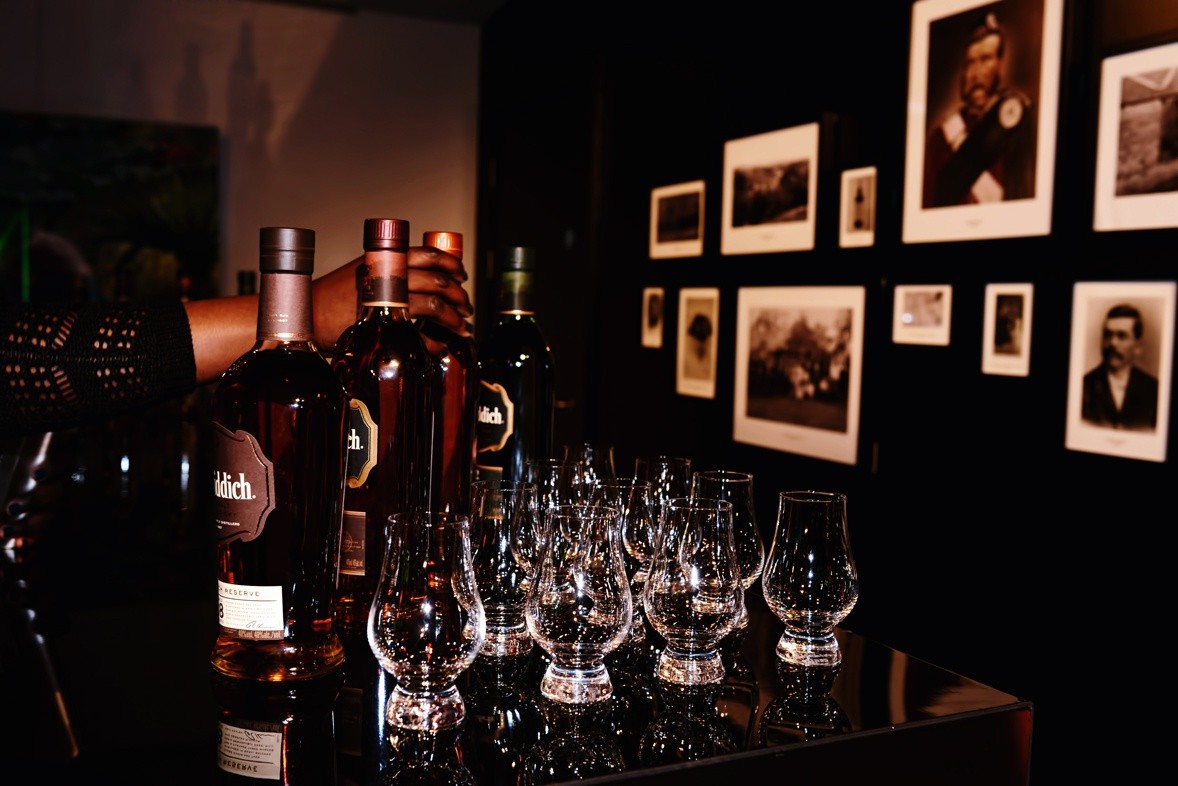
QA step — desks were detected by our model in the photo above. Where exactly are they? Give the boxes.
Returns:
[134,607,1035,785]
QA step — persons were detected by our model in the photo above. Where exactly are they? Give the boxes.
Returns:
[923,29,1036,207]
[1081,304,1159,433]
[0,244,474,404]
[684,316,712,381]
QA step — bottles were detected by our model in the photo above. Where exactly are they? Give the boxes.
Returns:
[207,637,347,786]
[210,226,346,646]
[411,228,476,576]
[20,419,198,555]
[329,215,434,641]
[472,245,557,544]
[71,269,254,307]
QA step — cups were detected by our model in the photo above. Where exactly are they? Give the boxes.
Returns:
[370,656,853,786]
[365,440,859,695]
